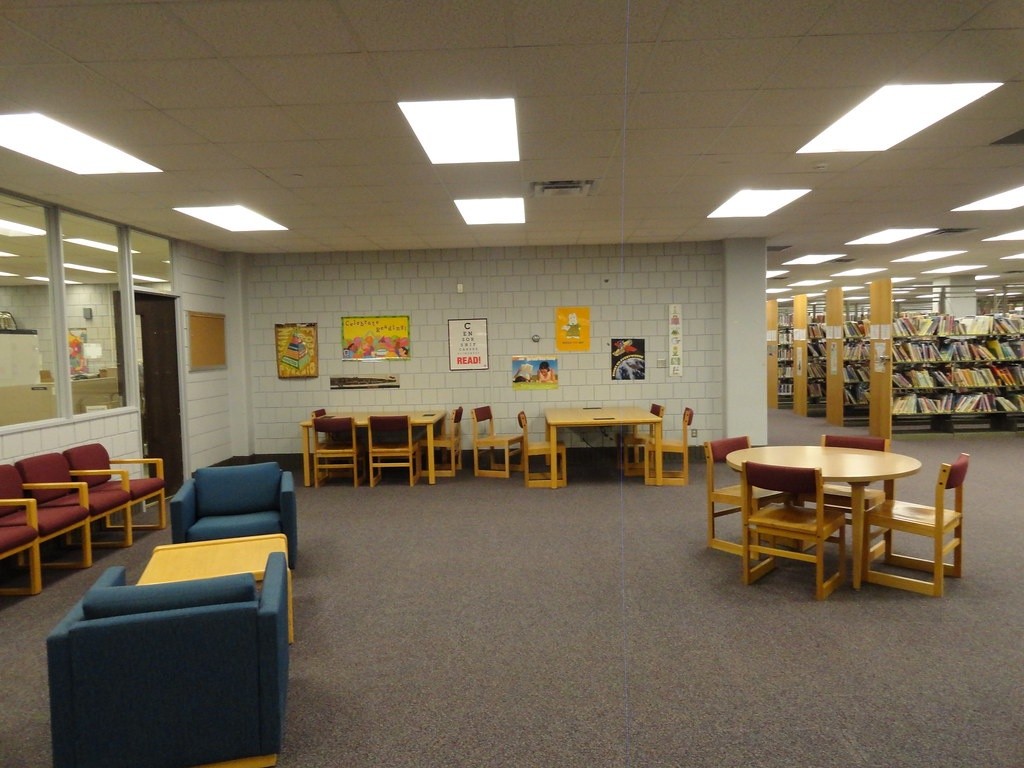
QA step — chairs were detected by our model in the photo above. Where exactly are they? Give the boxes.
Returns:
[304,404,969,600]
[0,444,168,597]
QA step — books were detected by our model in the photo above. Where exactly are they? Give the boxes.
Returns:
[776,309,1024,415]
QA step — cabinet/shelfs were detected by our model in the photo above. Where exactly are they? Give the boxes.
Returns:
[767,277,1024,439]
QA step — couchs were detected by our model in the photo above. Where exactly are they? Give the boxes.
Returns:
[45,552,289,768]
[169,462,298,571]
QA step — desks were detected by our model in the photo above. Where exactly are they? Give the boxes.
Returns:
[135,533,294,646]
[546,403,662,489]
[299,411,446,487]
[727,445,921,593]
